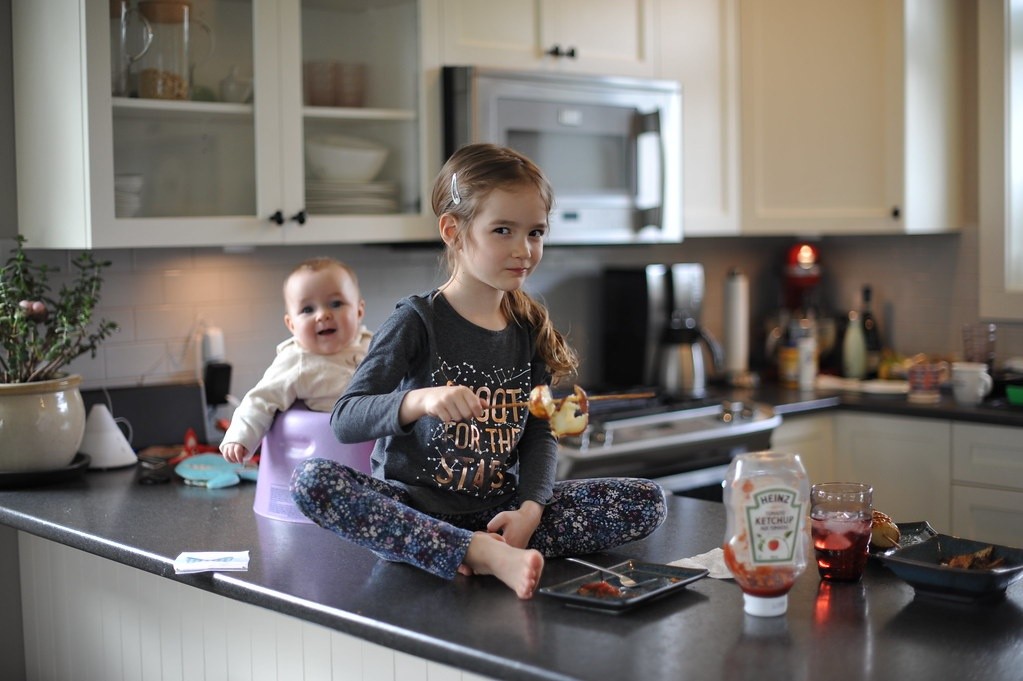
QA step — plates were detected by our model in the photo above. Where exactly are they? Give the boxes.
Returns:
[305,179,399,216]
[539,558,711,617]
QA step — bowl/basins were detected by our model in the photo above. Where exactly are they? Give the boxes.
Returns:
[306,57,369,107]
[114,171,145,216]
[306,130,388,184]
[868,521,1023,600]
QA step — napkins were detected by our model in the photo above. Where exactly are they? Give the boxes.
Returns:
[173,551,250,575]
[673,549,734,579]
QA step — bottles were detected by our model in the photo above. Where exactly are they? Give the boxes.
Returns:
[722,450,813,616]
[860,284,883,380]
[841,311,868,380]
[774,325,819,392]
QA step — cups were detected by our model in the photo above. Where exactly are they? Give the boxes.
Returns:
[950,361,993,405]
[961,322,997,370]
[907,359,951,402]
[109,1,215,102]
[809,481,875,586]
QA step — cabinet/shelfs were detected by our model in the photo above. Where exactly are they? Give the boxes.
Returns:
[367,0,644,77]
[645,1,738,236]
[831,406,949,536]
[11,1,441,249]
[949,420,1022,549]
[737,0,969,235]
[770,409,830,500]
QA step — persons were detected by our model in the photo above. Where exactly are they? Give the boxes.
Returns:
[288,143,667,599]
[218,258,374,464]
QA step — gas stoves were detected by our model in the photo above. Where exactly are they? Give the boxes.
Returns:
[546,379,782,486]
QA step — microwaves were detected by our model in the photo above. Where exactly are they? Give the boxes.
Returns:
[437,64,685,249]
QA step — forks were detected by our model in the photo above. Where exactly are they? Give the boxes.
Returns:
[567,557,658,587]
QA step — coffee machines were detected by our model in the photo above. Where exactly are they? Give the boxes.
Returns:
[596,260,723,404]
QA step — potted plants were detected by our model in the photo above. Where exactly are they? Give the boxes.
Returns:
[0,234,121,476]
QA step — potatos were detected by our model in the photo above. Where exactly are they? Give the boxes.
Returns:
[527,384,589,439]
[871,511,900,548]
